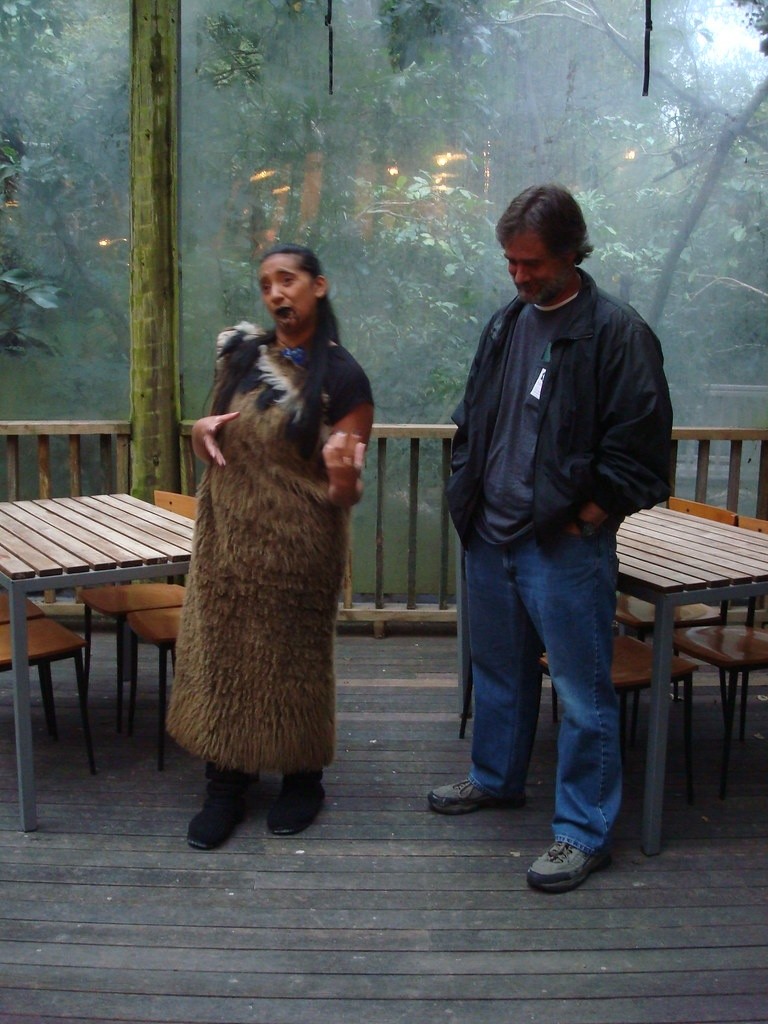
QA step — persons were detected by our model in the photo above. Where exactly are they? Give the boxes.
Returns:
[428,183,676,894]
[165,241,374,852]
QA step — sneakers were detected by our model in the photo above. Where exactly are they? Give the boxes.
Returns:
[526,842,600,892]
[426,779,526,814]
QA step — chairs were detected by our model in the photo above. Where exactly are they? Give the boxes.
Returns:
[77,490,198,736]
[539,631,698,805]
[0,589,57,739]
[454,547,558,741]
[602,498,737,721]
[666,514,768,800]
[0,617,97,778]
[125,606,183,772]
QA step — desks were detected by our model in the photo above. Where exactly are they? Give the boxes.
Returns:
[455,505,768,858]
[0,492,196,832]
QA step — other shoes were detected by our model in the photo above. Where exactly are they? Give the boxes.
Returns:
[187,782,252,849]
[267,778,324,834]
